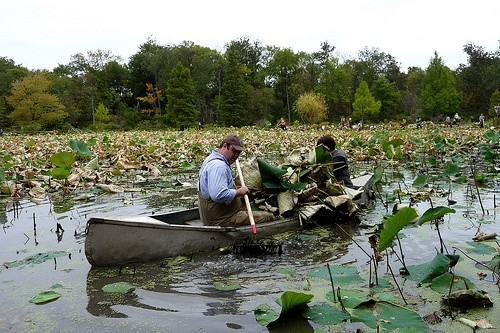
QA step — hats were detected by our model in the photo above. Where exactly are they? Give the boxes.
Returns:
[223,134,245,151]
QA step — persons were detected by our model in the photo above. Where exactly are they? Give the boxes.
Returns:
[317,137,353,188]
[281,112,485,130]
[197,133,276,226]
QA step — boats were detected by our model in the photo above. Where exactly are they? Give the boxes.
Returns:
[85,172,375,266]
